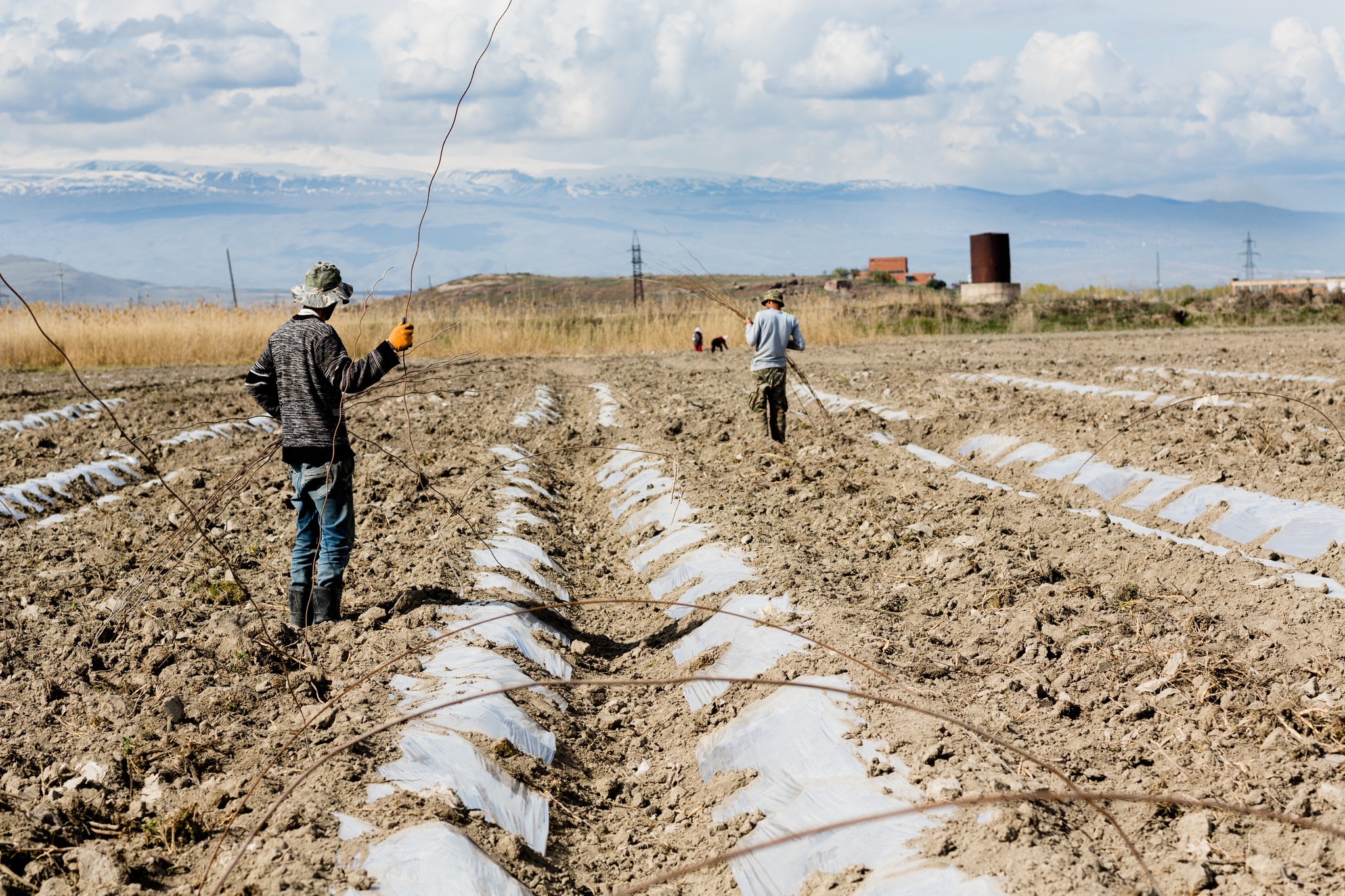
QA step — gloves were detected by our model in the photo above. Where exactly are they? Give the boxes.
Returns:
[388,317,414,352]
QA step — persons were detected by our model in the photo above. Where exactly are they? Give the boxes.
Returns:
[245,261,415,629]
[691,327,703,352]
[743,290,805,444]
[711,334,729,353]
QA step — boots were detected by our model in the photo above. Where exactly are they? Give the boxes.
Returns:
[311,586,343,626]
[288,581,314,629]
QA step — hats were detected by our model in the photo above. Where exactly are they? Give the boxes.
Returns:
[722,335,728,341]
[694,326,702,333]
[761,289,785,307]
[290,262,353,309]
